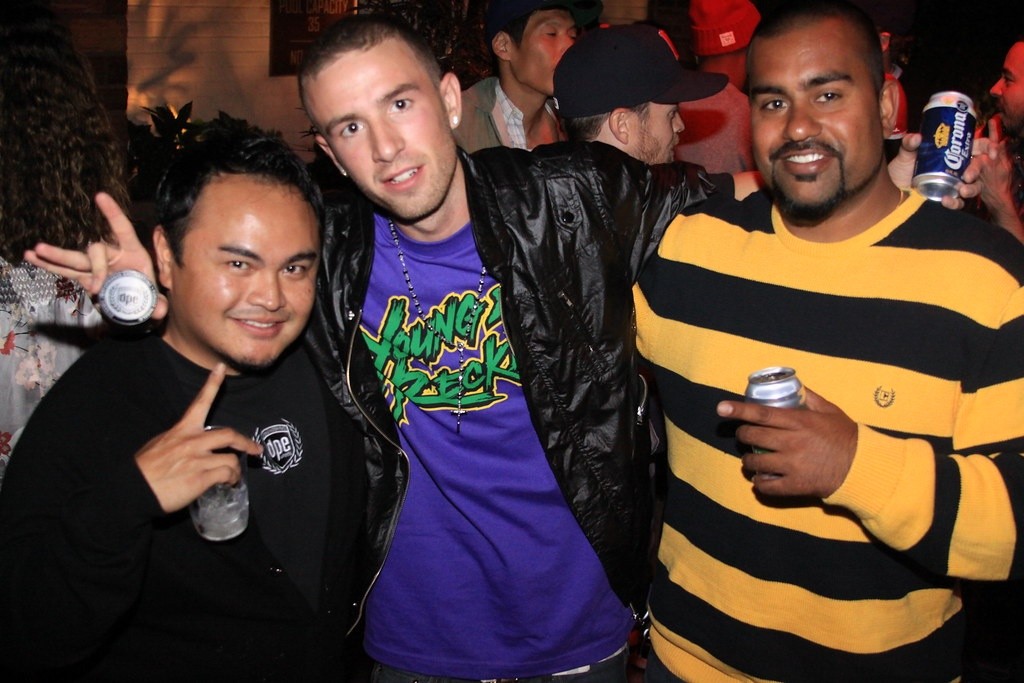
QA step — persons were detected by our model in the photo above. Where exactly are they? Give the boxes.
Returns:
[877,30,1024,247]
[0,0,132,473]
[674,1,760,175]
[552,23,729,165]
[453,0,604,153]
[632,0,1024,682]
[20,11,985,683]
[0,124,368,683]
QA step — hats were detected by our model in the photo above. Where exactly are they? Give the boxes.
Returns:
[553,24,729,119]
[483,0,604,43]
[689,0,761,56]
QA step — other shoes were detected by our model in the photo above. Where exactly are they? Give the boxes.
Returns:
[628,623,651,669]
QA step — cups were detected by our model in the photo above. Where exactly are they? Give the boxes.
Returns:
[191,427,249,541]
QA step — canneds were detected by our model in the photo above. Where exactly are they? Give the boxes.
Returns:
[910,90,979,202]
[745,366,808,478]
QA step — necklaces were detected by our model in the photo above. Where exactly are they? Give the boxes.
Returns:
[388,218,489,436]
